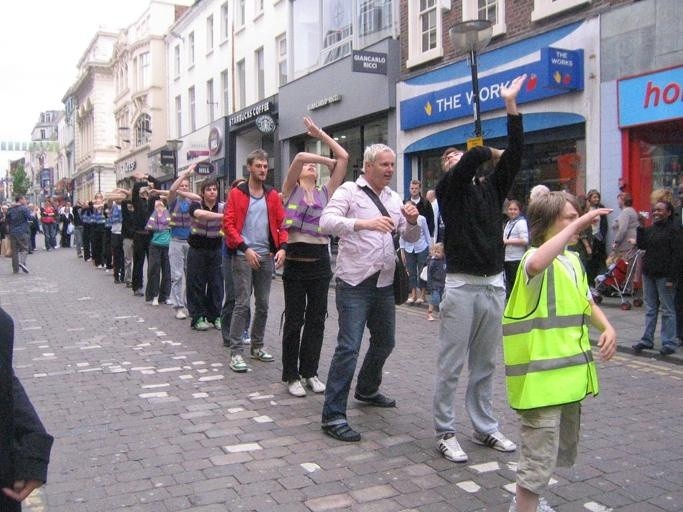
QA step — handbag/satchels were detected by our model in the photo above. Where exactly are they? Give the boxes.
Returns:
[394,261,408,305]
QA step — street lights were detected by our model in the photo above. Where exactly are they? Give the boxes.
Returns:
[94,164,103,192]
[166,138,183,181]
[448,16,492,147]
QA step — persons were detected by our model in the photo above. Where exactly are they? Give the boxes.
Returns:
[504,184,608,311]
[74,162,253,347]
[317,143,423,443]
[223,147,289,374]
[0,311,54,512]
[403,179,446,320]
[279,114,350,397]
[432,70,526,464]
[1,195,74,273]
[607,187,683,355]
[502,187,617,512]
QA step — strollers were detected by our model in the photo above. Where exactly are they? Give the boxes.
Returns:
[587,242,647,312]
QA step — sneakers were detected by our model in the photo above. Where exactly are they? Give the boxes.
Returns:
[632,343,652,350]
[436,434,468,462]
[661,347,675,355]
[354,391,395,407]
[471,430,517,451]
[509,495,555,512]
[321,424,360,442]
